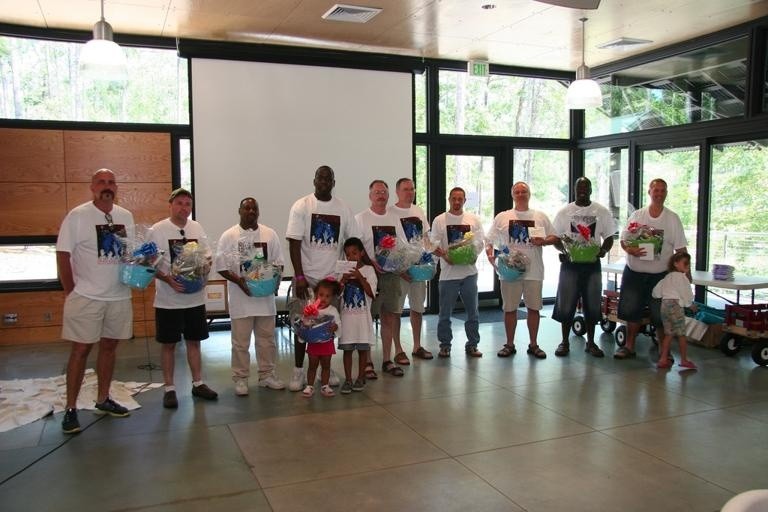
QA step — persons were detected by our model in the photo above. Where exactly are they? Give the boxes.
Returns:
[355,180,410,380]
[484,181,557,360]
[613,178,688,360]
[285,165,381,392]
[335,236,378,395]
[299,276,341,399]
[143,188,218,408]
[392,178,433,365]
[215,197,285,396]
[54,167,136,436]
[651,251,698,368]
[429,187,484,357]
[553,176,618,358]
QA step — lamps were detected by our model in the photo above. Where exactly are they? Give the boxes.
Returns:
[275,278,295,326]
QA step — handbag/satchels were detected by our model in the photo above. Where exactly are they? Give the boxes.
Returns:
[295,274,306,282]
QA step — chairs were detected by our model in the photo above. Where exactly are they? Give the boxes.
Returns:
[163,390,178,408]
[94,396,129,417]
[235,380,249,396]
[555,342,569,356]
[289,370,306,391]
[62,407,81,433]
[257,377,285,390]
[192,383,218,400]
[584,342,604,357]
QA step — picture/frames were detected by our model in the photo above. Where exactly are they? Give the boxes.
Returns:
[595,262,768,304]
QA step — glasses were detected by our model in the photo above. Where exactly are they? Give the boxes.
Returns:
[75,0,128,65]
[563,15,604,112]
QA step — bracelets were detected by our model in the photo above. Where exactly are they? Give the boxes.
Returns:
[379,236,660,281]
[116,260,159,290]
[296,313,332,344]
[174,271,208,294]
[244,270,280,298]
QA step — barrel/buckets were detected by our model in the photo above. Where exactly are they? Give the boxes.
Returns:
[439,349,450,357]
[412,347,433,359]
[679,361,697,368]
[394,352,410,365]
[613,347,636,358]
[471,350,482,356]
[657,360,672,368]
[667,353,674,363]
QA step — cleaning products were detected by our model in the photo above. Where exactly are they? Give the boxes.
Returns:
[721,302,768,366]
[571,286,658,347]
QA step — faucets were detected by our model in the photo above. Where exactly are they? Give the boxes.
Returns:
[527,344,546,358]
[498,344,516,357]
[303,361,404,397]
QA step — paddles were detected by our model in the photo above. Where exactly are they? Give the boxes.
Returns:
[447,308,547,323]
